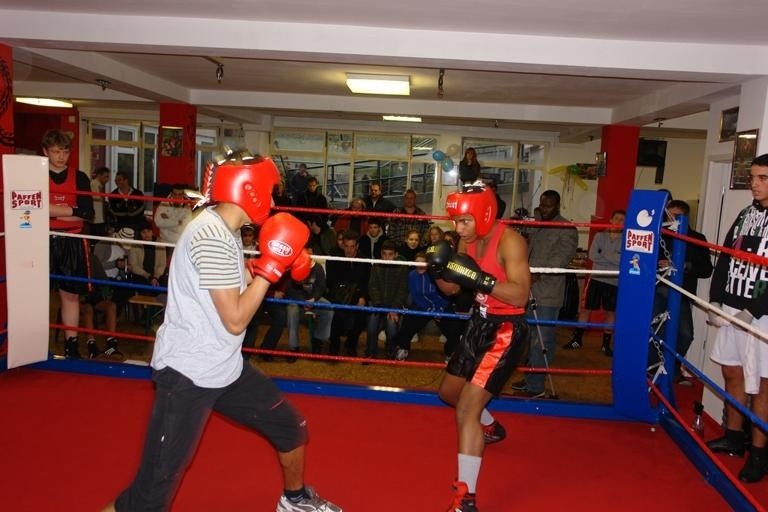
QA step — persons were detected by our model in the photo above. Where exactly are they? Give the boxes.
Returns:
[362,180,397,226]
[333,197,369,239]
[42,129,94,362]
[439,230,473,343]
[512,189,579,402]
[347,217,389,347]
[155,185,191,250]
[460,146,480,188]
[706,153,768,484]
[303,214,323,256]
[486,184,507,221]
[273,179,291,207]
[241,279,288,362]
[288,163,311,207]
[286,247,335,362]
[95,227,136,329]
[510,209,529,239]
[107,172,152,238]
[87,167,111,231]
[424,180,531,512]
[97,222,168,330]
[326,234,373,354]
[162,131,181,156]
[359,240,410,366]
[327,231,350,269]
[92,155,344,511]
[294,176,328,255]
[395,251,462,363]
[388,190,430,253]
[240,225,260,259]
[378,231,425,341]
[425,225,444,252]
[648,201,713,388]
[79,235,126,366]
[561,210,626,358]
[533,206,544,222]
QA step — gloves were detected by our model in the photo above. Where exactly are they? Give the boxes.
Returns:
[247,247,310,284]
[444,251,495,293]
[251,211,310,283]
[426,239,453,279]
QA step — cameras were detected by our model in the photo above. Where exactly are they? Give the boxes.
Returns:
[505,206,537,234]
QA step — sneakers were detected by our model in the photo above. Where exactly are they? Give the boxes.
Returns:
[601,343,614,357]
[410,333,418,342]
[678,374,692,387]
[310,340,321,356]
[63,339,84,361]
[104,340,127,362]
[87,342,104,359]
[393,348,409,361]
[561,339,584,351]
[288,349,298,362]
[738,456,768,483]
[706,437,744,458]
[259,343,271,360]
[362,352,374,363]
[383,350,395,364]
[444,494,477,511]
[445,352,455,364]
[514,391,546,399]
[482,421,506,445]
[378,333,386,341]
[510,378,527,388]
[275,489,343,512]
[440,334,447,343]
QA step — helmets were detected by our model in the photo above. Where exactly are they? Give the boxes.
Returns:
[443,178,498,239]
[210,150,282,226]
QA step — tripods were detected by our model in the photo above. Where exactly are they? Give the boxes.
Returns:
[514,233,559,401]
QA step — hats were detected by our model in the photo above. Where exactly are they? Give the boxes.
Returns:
[117,227,135,250]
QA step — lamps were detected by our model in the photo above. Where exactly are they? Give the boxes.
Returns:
[383,115,422,122]
[346,74,409,95]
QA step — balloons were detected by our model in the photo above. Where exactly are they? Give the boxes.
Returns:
[433,151,444,161]
[446,144,459,156]
[442,159,454,171]
